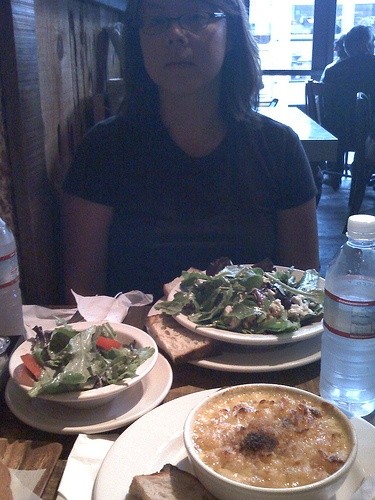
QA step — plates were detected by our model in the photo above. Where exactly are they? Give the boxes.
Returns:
[4,353,175,435]
[146,296,321,374]
[9,321,159,407]
[91,387,375,500]
[167,264,325,346]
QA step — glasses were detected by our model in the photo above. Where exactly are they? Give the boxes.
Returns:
[138,10,229,37]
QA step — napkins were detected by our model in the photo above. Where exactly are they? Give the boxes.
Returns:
[70,290,153,332]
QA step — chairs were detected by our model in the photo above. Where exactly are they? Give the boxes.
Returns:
[256,79,375,233]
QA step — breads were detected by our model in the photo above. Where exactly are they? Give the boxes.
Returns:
[146,312,224,363]
[161,267,200,297]
[128,463,220,500]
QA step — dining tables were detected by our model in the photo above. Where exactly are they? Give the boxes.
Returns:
[0,306,375,500]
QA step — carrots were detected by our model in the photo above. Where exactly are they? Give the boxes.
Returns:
[20,353,41,380]
[96,336,122,350]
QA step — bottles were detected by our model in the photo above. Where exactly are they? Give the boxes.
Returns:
[320,214,375,417]
[0,216,29,340]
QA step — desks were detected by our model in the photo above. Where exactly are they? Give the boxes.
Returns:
[255,107,337,208]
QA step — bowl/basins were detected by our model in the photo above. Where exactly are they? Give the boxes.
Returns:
[184,382,359,500]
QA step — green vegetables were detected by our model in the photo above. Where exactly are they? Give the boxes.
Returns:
[155,255,325,336]
[21,314,153,398]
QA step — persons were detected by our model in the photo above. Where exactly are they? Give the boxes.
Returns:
[319,25,375,192]
[59,0,319,307]
